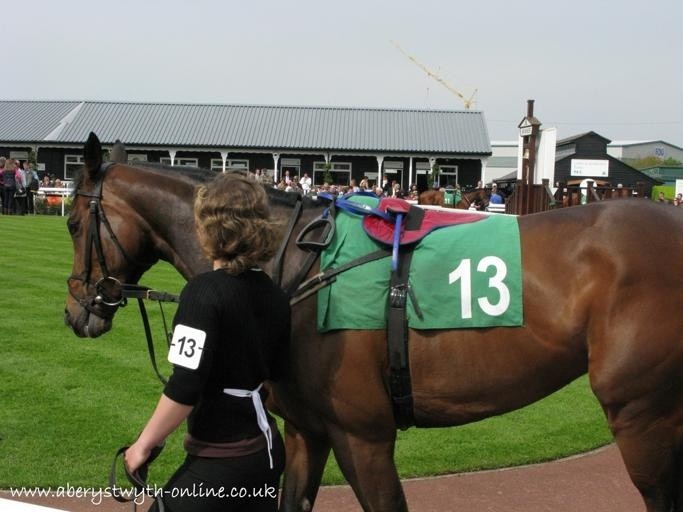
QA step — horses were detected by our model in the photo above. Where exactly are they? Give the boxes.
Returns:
[61,128,683,512]
[416,186,493,213]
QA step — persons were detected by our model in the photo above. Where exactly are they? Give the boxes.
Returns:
[123,169,291,512]
[0,157,683,216]
